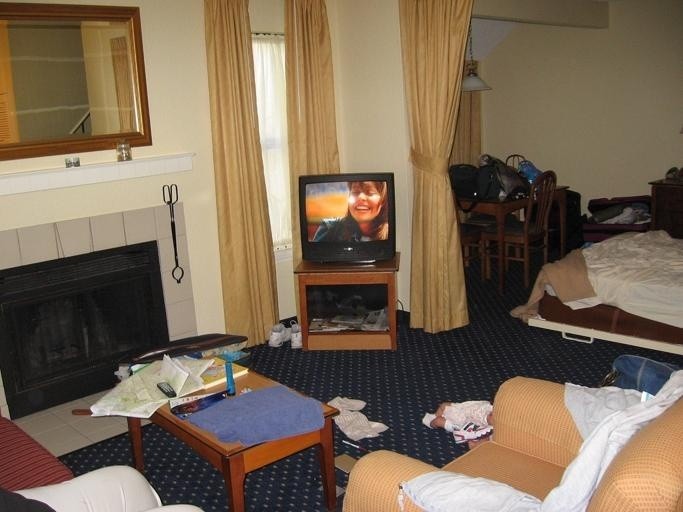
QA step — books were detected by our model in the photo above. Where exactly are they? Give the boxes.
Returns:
[192,355,248,389]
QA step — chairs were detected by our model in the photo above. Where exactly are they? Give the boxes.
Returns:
[452,153,557,298]
[341,367,682,511]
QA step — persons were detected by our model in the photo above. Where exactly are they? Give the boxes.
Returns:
[313,181,389,242]
[430,400,492,444]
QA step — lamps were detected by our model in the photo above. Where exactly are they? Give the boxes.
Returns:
[458,15,493,93]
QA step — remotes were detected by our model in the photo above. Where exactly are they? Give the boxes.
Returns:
[155,378,177,398]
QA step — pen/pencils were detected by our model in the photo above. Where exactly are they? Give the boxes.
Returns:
[342,439,370,454]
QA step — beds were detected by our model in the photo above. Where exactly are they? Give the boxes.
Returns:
[527,231,683,357]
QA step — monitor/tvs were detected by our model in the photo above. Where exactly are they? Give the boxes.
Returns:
[298,171,396,266]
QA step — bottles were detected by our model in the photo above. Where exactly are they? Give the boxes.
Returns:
[116,143,132,161]
[65,156,80,168]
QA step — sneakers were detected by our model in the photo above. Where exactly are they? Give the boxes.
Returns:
[290,324,303,349]
[268,323,290,348]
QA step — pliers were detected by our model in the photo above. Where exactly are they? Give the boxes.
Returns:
[163,184,184,283]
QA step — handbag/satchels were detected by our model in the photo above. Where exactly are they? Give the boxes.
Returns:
[448,154,543,203]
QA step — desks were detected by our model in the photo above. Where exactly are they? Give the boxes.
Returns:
[454,183,570,297]
[292,252,404,352]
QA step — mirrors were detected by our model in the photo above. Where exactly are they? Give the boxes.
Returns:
[0,1,152,162]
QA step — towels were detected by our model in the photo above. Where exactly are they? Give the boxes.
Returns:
[185,385,328,451]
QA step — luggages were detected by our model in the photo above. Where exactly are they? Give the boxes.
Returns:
[583,195,651,242]
